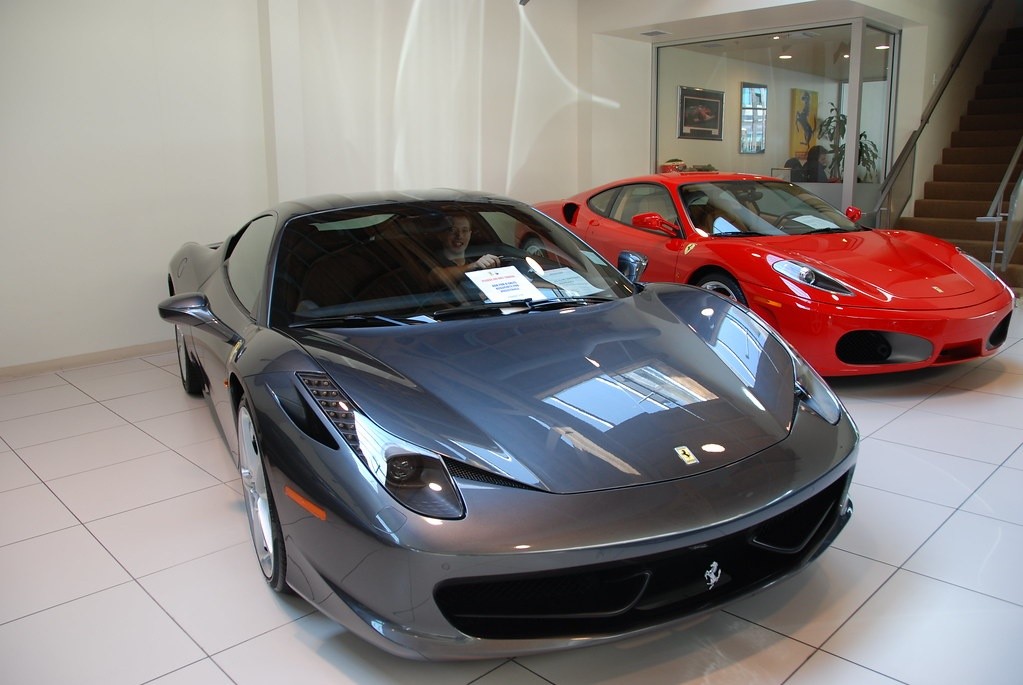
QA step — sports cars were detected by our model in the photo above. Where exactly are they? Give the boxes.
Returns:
[157,188,859,662]
[531,172,1015,377]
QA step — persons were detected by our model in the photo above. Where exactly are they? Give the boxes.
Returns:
[803,146,839,183]
[783,158,803,182]
[407,211,501,291]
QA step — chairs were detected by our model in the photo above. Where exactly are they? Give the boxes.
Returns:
[297,244,426,312]
[624,196,676,237]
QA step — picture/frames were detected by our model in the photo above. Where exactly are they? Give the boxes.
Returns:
[675,86,726,141]
